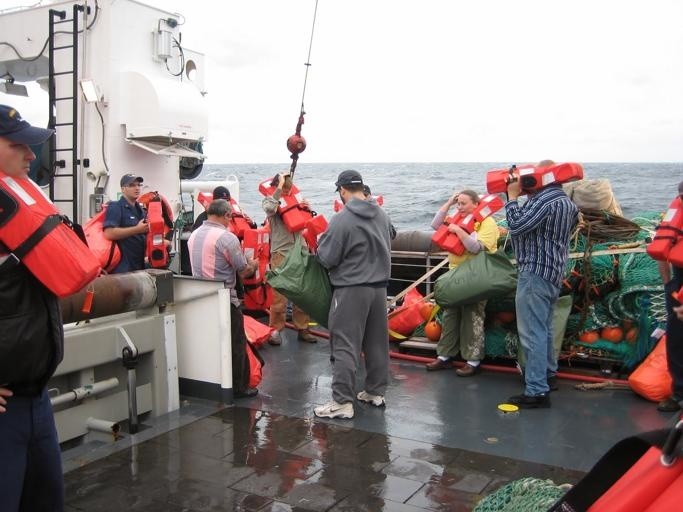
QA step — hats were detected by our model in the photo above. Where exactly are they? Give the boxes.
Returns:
[121,173,143,186]
[213,187,230,200]
[335,171,371,196]
[0,105,55,146]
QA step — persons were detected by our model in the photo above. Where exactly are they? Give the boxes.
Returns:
[504,159,577,407]
[1,103,66,510]
[656,183,682,412]
[261,172,318,344]
[363,184,371,199]
[424,189,498,375]
[315,170,392,419]
[103,173,149,273]
[187,199,261,398]
[188,187,258,309]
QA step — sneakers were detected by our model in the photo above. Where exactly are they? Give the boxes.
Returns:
[509,376,557,409]
[357,391,385,406]
[314,399,354,419]
[456,363,480,376]
[269,330,317,345]
[426,358,449,370]
[658,397,683,411]
